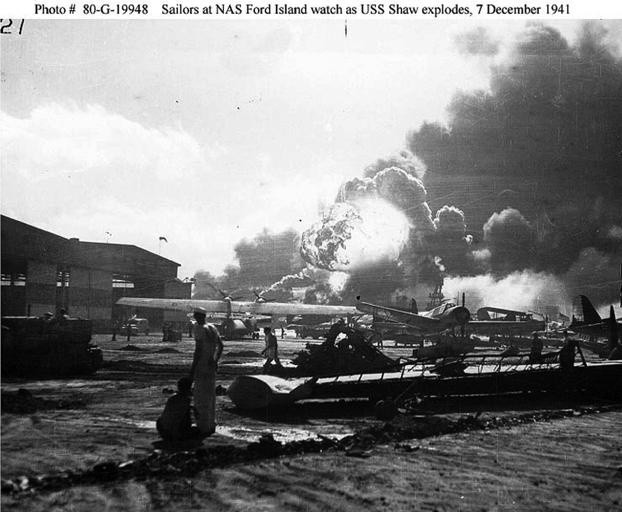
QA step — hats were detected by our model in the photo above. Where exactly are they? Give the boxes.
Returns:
[194,306,207,314]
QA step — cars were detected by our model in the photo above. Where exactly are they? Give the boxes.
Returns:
[120,318,149,335]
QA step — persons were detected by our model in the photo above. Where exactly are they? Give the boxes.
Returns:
[161,323,168,342]
[189,306,223,438]
[189,321,193,337]
[559,331,573,369]
[111,320,119,341]
[155,376,199,441]
[259,326,284,375]
[529,331,543,364]
[127,323,131,341]
